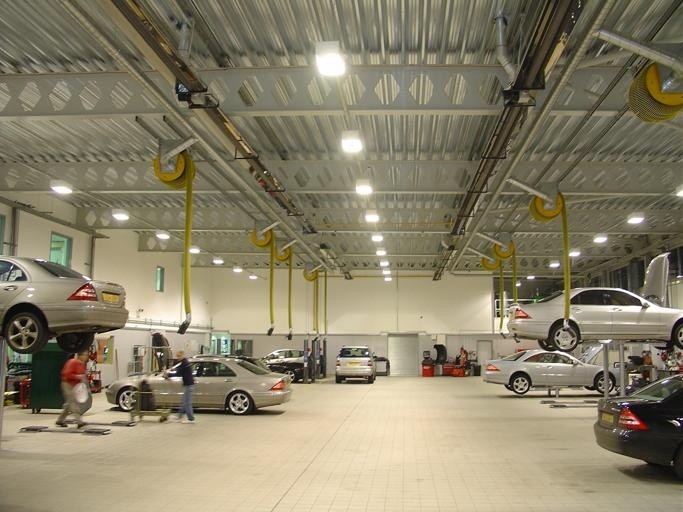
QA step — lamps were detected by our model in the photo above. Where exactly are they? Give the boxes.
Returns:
[356,178,373,195]
[548,211,645,268]
[365,209,392,282]
[315,41,346,77]
[49,179,243,273]
[341,130,362,153]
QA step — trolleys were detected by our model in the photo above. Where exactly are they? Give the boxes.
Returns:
[129,378,172,422]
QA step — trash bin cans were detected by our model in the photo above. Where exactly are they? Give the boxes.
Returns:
[374,358,387,372]
[422,359,434,377]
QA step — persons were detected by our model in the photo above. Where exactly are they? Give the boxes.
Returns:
[149,330,172,370]
[54,348,93,430]
[162,350,196,424]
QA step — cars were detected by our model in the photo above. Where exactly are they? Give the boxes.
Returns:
[335,345,375,384]
[482,340,625,395]
[593,374,682,481]
[105,349,303,416]
[506,251,682,352]
[0,255,129,354]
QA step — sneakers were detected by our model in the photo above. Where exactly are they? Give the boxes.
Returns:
[54,419,86,429]
[169,415,197,424]
